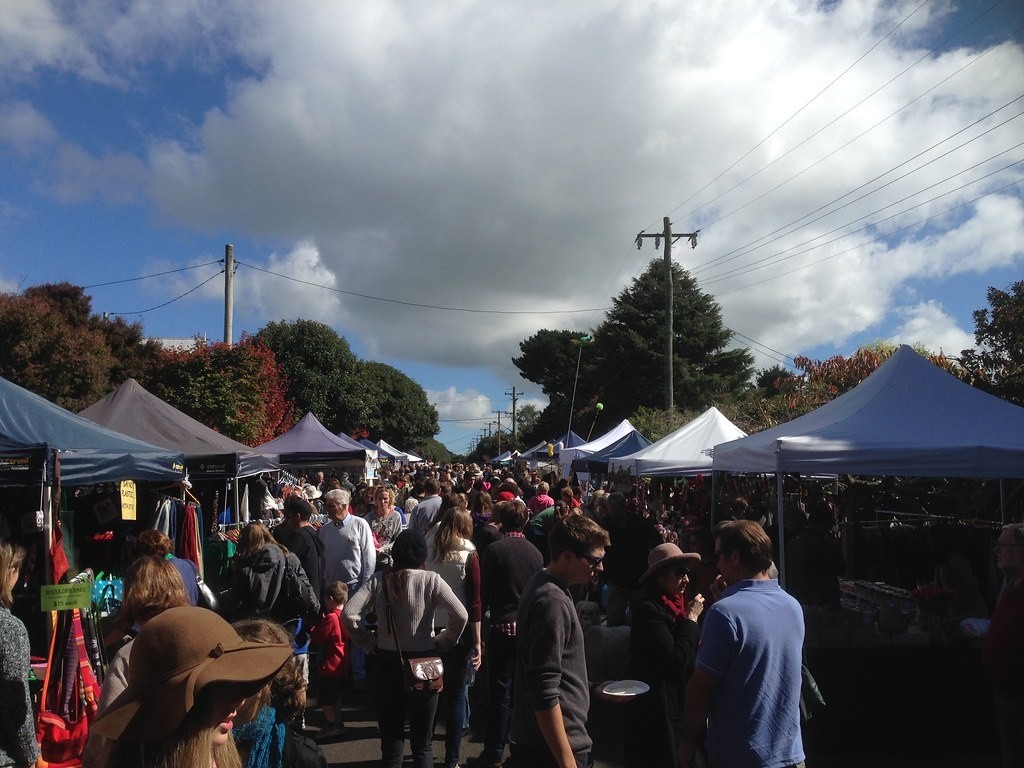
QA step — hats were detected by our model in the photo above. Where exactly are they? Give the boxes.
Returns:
[280,500,312,516]
[304,485,323,500]
[330,478,340,485]
[89,605,295,743]
[464,472,478,479]
[639,542,701,585]
[496,492,516,502]
[290,486,303,499]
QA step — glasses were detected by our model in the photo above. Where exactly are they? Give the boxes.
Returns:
[713,550,726,563]
[663,568,691,578]
[570,550,606,568]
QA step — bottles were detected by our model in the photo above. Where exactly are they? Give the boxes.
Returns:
[463,649,476,686]
[838,577,917,628]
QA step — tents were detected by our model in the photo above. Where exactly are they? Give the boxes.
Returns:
[248,409,386,474]
[555,419,655,490]
[608,405,839,523]
[76,378,283,529]
[358,435,425,469]
[709,345,1024,617]
[487,431,589,470]
[0,375,188,546]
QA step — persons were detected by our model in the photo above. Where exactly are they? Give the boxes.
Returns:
[423,504,485,768]
[362,485,403,569]
[228,517,325,729]
[318,487,378,689]
[314,580,353,744]
[1,461,1023,768]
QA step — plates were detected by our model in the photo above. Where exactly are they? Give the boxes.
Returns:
[603,680,650,696]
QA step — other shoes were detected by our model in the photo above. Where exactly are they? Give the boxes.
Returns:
[313,720,345,740]
[456,757,504,768]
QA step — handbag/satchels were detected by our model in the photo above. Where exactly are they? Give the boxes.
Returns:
[31,699,88,768]
[404,659,443,693]
